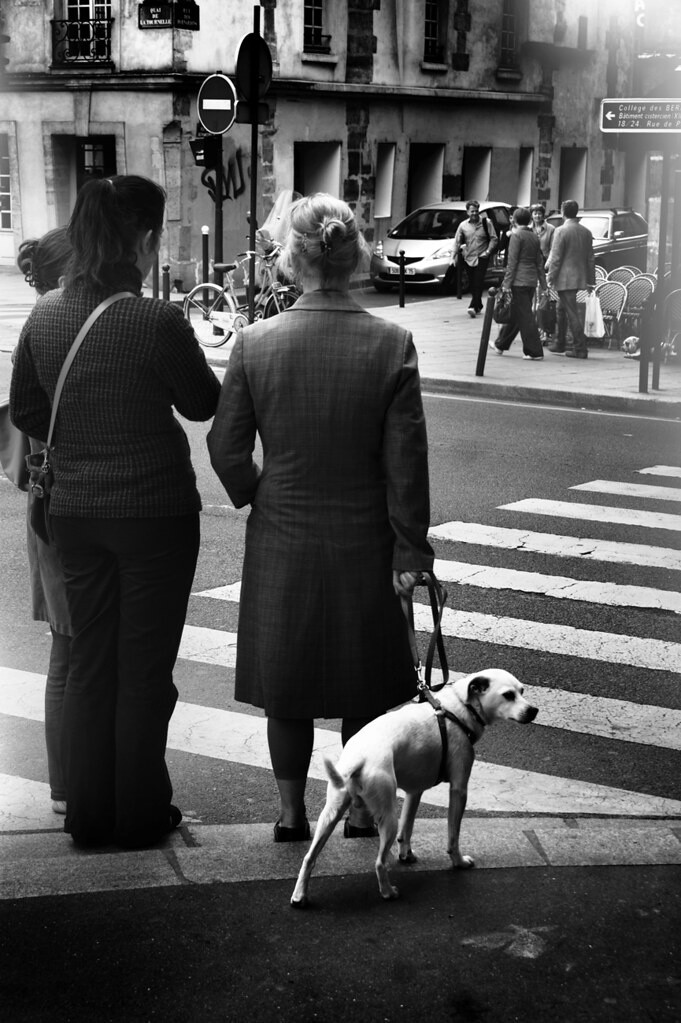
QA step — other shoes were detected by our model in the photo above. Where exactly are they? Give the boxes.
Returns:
[467,308,476,318]
[273,816,310,842]
[50,798,68,812]
[548,343,563,352]
[487,341,504,354]
[343,816,378,838]
[565,349,587,358]
[524,354,543,360]
[66,804,182,849]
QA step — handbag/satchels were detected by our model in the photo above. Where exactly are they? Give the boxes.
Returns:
[537,289,556,334]
[583,289,606,338]
[24,448,55,499]
[492,292,514,323]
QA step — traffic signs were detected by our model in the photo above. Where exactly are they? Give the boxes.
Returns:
[599,98,679,135]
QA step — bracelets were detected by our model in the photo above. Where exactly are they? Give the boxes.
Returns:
[486,250,491,254]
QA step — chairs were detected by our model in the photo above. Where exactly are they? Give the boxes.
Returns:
[576,263,681,364]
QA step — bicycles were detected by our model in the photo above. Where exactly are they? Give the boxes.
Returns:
[180,249,305,347]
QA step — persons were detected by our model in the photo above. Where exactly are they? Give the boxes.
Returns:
[494,207,520,273]
[17,227,72,814]
[489,208,550,360]
[449,200,498,317]
[206,192,435,842]
[529,204,556,273]
[9,175,221,854]
[547,200,597,359]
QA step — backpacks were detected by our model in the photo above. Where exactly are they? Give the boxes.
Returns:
[482,217,500,254]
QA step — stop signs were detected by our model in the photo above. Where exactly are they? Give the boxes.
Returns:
[197,74,239,137]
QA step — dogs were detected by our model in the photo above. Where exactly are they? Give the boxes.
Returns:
[621,335,678,359]
[290,667,539,907]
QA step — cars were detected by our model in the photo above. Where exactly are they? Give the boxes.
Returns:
[367,201,534,288]
[537,209,651,279]
